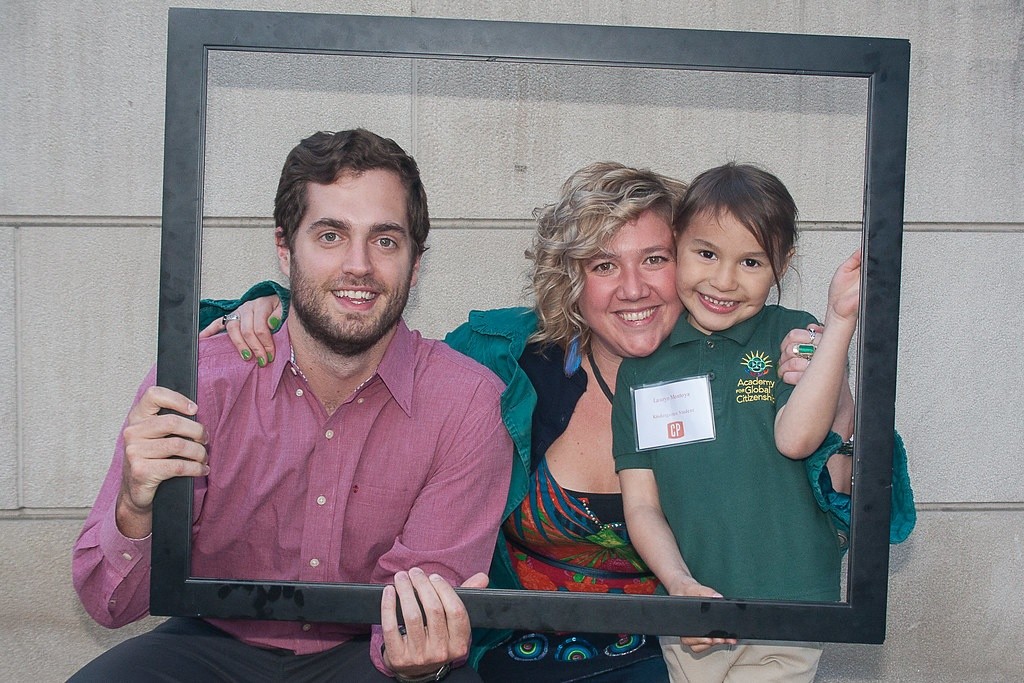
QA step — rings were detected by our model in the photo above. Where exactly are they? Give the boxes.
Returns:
[221,315,241,325]
[793,343,817,361]
[808,328,816,344]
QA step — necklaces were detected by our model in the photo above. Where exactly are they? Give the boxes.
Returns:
[586,341,614,405]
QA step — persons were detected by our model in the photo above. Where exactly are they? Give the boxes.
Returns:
[199,161,917,683]
[65,128,513,683]
[610,157,862,683]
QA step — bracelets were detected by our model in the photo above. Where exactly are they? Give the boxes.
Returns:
[381,627,453,683]
